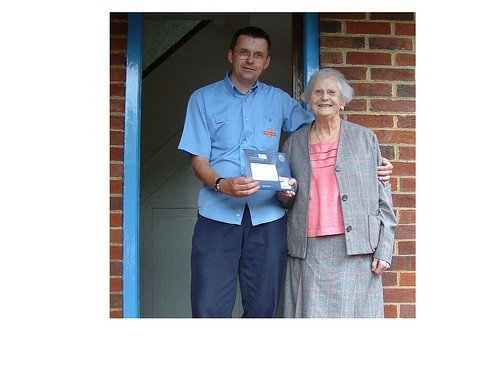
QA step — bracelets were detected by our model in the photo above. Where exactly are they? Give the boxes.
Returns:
[213,177,226,194]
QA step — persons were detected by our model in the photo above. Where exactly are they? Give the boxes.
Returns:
[177,25,393,318]
[276,68,397,319]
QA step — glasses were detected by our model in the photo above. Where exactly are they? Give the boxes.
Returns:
[233,49,270,60]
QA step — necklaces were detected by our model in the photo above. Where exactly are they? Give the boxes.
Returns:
[313,123,338,143]
